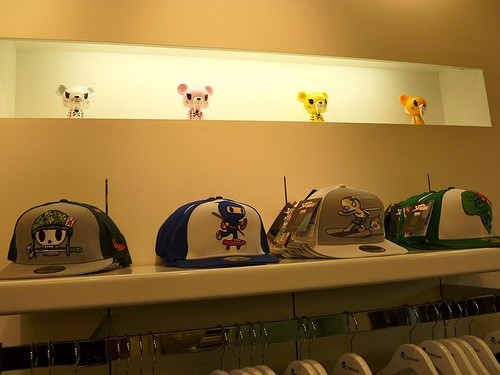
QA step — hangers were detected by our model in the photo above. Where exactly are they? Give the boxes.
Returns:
[30,296,500,375]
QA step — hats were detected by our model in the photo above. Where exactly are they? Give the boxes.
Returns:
[384,187,500,251]
[0,198,134,279]
[154,195,279,268]
[269,183,409,260]
